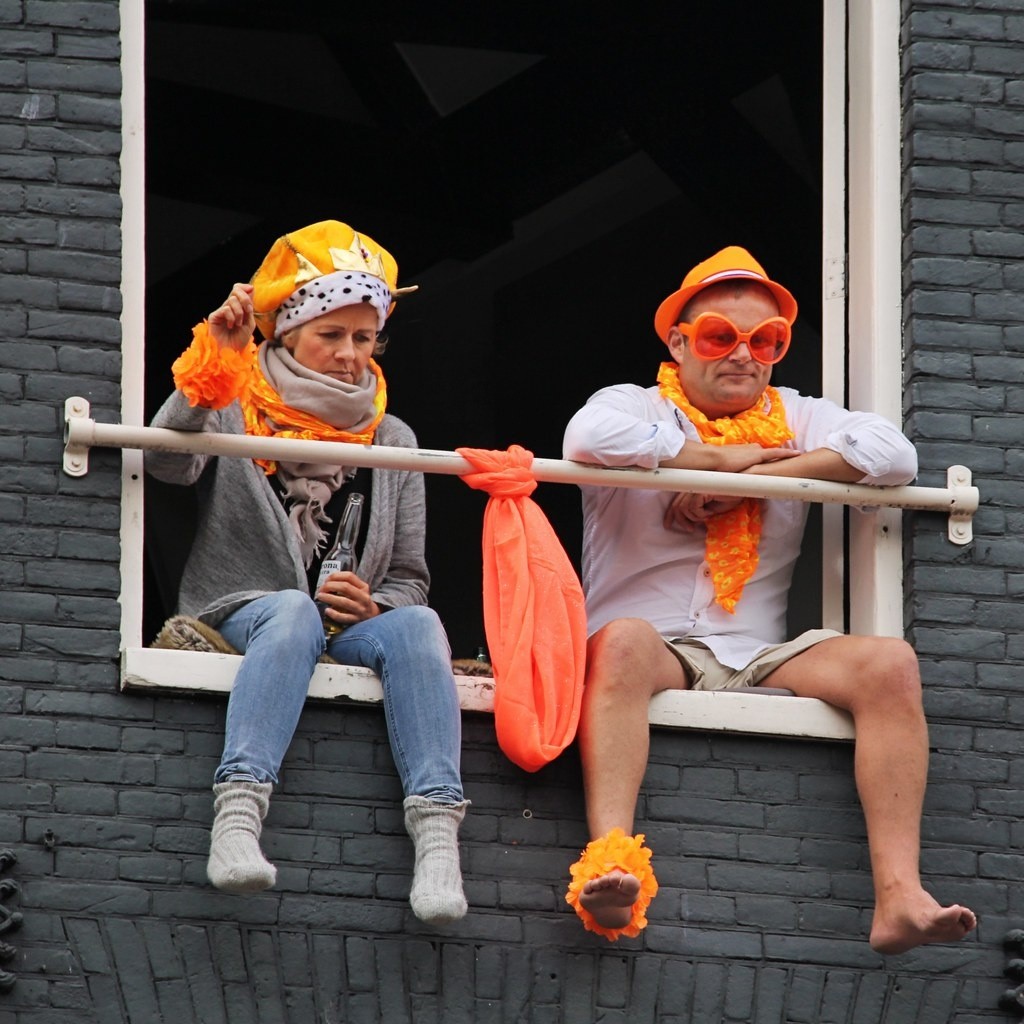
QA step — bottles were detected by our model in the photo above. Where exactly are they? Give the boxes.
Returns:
[313,493,365,634]
[476,647,487,664]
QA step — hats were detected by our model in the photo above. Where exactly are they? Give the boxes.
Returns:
[653,246,798,345]
[248,217,418,342]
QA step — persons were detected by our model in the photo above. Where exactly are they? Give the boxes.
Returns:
[143,220,469,925]
[561,246,978,955]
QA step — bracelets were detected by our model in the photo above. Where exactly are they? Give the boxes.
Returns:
[170,315,257,409]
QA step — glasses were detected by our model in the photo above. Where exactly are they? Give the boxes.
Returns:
[680,312,793,365]
